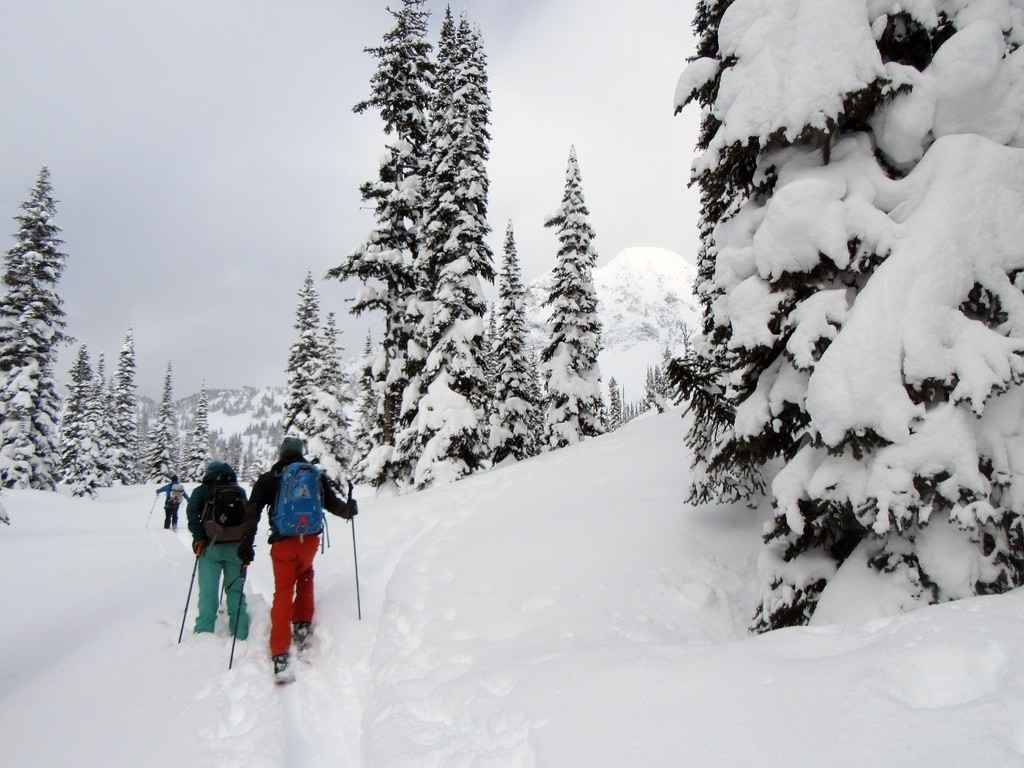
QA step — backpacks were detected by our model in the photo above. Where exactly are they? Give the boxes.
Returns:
[202,483,249,542]
[271,462,323,536]
[168,483,184,506]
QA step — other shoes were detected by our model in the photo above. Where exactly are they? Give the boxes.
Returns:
[292,622,310,642]
[272,654,294,683]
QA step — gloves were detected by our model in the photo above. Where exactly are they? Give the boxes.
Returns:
[345,499,358,519]
[237,543,254,566]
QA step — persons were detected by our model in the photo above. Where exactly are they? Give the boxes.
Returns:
[238,438,357,683]
[156,475,190,530]
[186,461,251,640]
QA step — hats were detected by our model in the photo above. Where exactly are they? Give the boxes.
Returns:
[280,437,302,456]
[171,475,178,481]
[207,460,222,474]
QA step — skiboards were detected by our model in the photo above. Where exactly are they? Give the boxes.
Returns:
[272,634,319,685]
